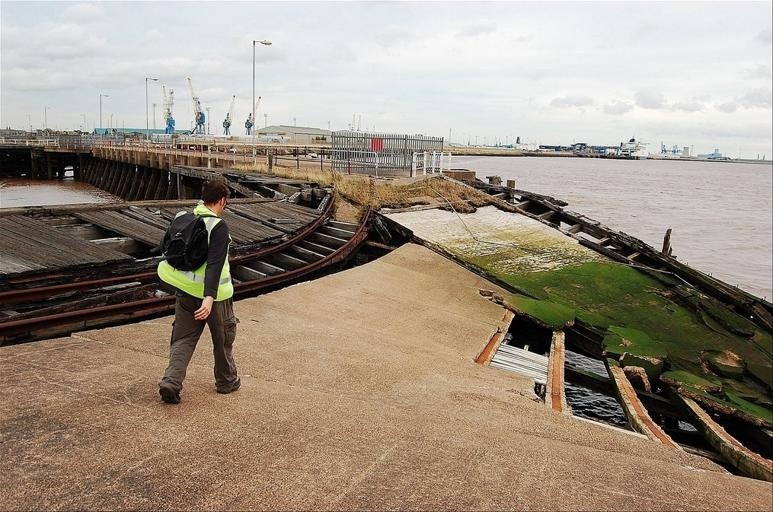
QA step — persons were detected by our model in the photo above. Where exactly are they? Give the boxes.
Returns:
[157,179,239,405]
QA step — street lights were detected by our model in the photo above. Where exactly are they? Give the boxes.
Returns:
[292,118,296,126]
[98,94,109,147]
[328,120,331,129]
[144,77,158,151]
[263,113,266,127]
[152,103,157,131]
[205,105,211,134]
[250,39,272,166]
[43,105,50,129]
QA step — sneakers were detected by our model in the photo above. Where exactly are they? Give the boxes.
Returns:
[217,376,240,393]
[159,385,181,403]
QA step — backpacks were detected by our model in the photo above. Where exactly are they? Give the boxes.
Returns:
[161,206,220,271]
